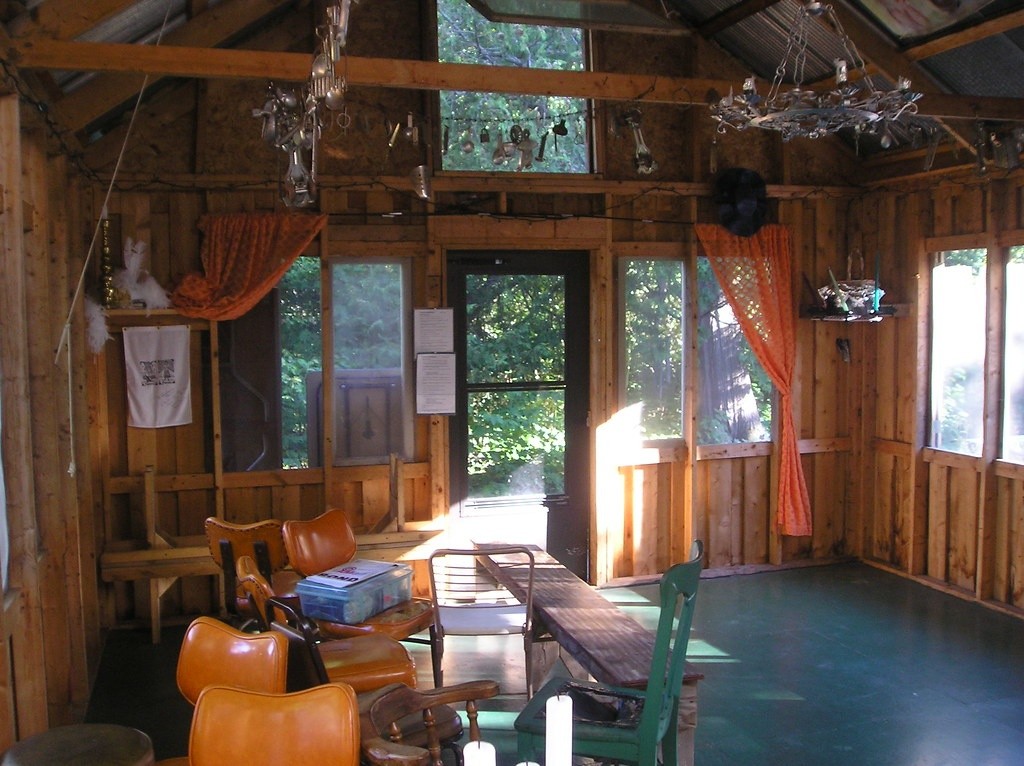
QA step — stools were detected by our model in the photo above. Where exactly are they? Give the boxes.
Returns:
[0,724,156,766]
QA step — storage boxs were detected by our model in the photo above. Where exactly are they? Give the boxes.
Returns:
[295,558,414,624]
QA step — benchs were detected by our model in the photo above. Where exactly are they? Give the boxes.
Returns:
[472,537,704,766]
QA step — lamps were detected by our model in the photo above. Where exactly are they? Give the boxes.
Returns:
[708,0,937,150]
[629,120,659,175]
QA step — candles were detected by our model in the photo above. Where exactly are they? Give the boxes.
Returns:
[827,267,853,316]
[545,688,572,766]
[516,759,540,766]
[462,736,497,766]
[873,251,880,311]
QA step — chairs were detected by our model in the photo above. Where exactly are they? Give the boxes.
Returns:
[267,595,464,766]
[188,682,361,766]
[156,616,288,766]
[237,556,417,695]
[429,547,535,702]
[358,680,499,766]
[515,537,705,766]
[284,508,441,689]
[205,517,288,633]
